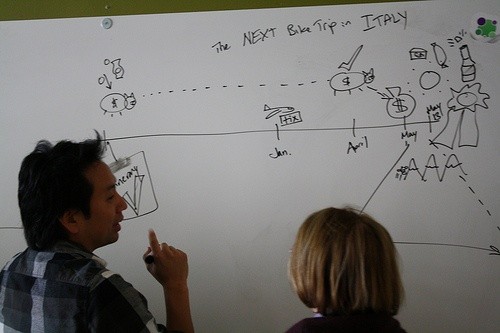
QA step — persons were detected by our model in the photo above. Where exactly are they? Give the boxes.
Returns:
[1,128,195,333]
[284,207,407,332]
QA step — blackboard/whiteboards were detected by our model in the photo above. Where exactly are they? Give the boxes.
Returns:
[0,0,500,333]
